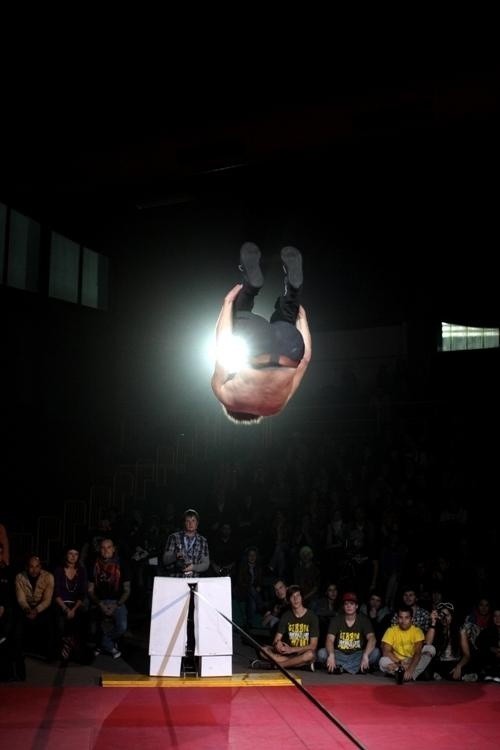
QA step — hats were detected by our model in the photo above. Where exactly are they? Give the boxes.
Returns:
[183,508,200,518]
[342,592,358,603]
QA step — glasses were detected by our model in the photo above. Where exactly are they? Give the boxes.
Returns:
[435,602,454,610]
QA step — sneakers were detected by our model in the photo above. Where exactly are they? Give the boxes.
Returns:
[251,659,275,670]
[301,661,316,673]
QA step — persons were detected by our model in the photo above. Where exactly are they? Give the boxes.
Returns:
[211,242,312,424]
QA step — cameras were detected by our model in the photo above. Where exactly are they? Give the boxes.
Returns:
[435,612,446,621]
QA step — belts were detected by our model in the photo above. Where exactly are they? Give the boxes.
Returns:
[248,355,301,368]
